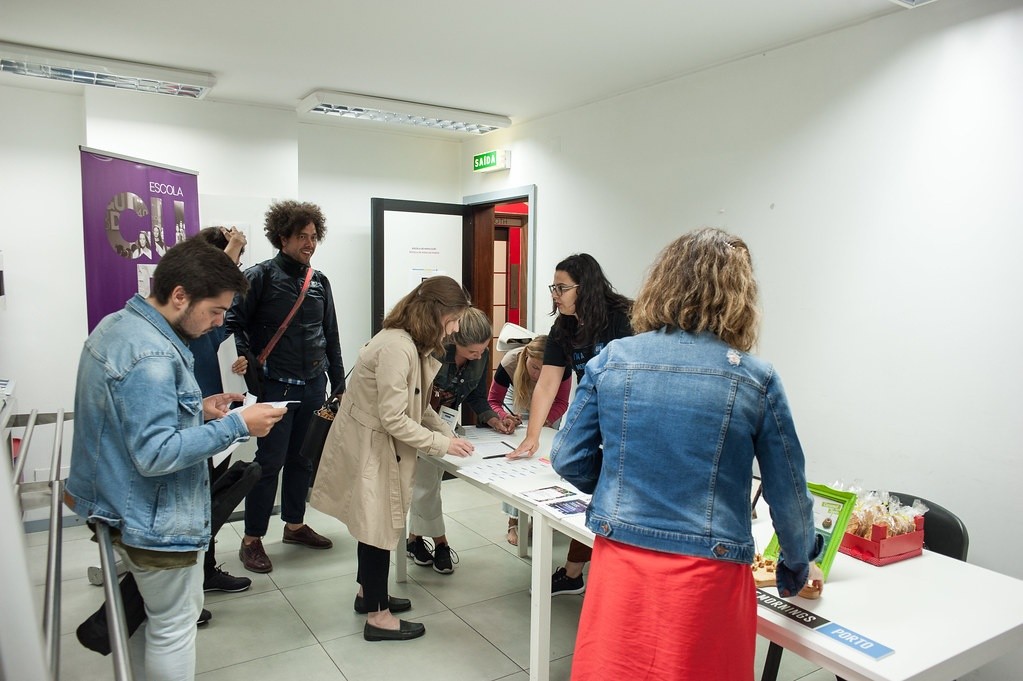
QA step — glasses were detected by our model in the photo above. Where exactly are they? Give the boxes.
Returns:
[548,284,579,296]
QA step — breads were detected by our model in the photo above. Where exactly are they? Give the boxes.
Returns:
[845,509,916,540]
[798,585,820,598]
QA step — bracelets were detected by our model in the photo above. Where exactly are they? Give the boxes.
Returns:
[494,419,500,431]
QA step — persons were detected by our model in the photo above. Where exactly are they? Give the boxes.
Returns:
[189,226,263,624]
[220,200,346,573]
[549,228,824,681]
[310,276,473,642]
[487,334,572,545]
[62,237,288,681]
[114,221,187,257]
[506,254,634,597]
[407,295,515,573]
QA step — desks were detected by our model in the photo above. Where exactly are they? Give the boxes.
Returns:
[395,420,1023,681]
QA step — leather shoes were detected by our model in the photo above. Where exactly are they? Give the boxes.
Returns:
[239,538,273,573]
[283,524,332,550]
[363,618,425,641]
[354,593,411,614]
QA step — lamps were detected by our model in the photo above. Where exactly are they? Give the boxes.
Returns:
[0,41,217,101]
[302,89,512,136]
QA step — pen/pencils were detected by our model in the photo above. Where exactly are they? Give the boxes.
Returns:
[500,400,524,425]
[483,455,505,459]
[501,441,515,450]
[752,482,763,510]
[451,430,457,438]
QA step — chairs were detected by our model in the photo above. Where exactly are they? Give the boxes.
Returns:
[761,490,969,681]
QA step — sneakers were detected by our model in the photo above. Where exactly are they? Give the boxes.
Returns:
[529,567,586,598]
[197,609,212,624]
[406,536,459,573]
[203,562,252,592]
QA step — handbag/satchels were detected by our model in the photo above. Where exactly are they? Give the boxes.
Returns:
[298,408,334,473]
[496,322,541,352]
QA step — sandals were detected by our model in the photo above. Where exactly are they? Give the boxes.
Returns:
[507,524,519,546]
